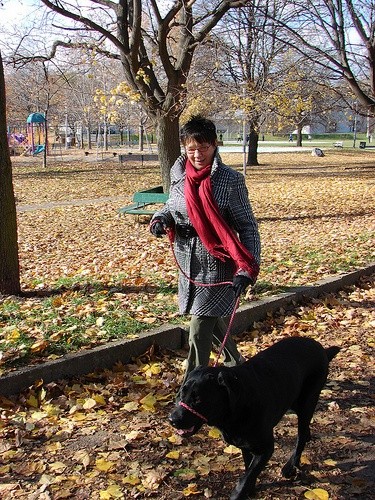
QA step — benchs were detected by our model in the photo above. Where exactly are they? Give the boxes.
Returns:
[117,185,169,223]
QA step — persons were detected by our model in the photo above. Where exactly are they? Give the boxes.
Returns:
[262,134,264,140]
[289,134,293,141]
[238,133,241,141]
[150,114,261,401]
[246,134,249,143]
[219,133,223,140]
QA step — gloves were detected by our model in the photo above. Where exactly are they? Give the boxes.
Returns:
[150,217,167,238]
[226,275,252,299]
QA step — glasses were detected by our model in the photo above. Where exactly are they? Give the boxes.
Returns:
[186,144,212,154]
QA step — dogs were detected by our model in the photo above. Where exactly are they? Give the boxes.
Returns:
[166,336,343,500]
